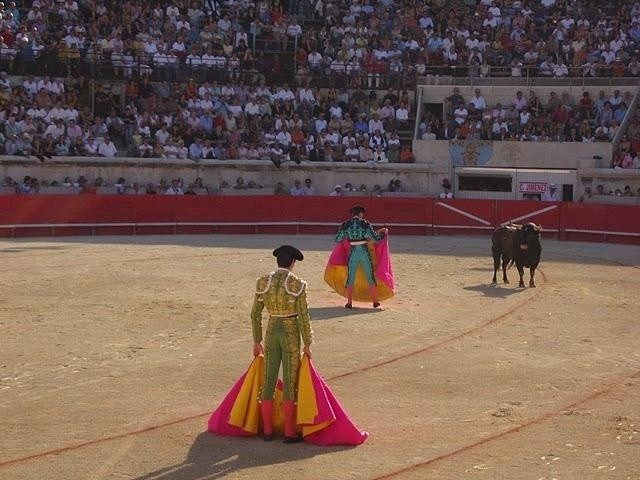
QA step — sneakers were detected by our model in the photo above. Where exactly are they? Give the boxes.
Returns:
[283,434,303,444]
[345,304,352,308]
[263,435,272,441]
[374,302,380,307]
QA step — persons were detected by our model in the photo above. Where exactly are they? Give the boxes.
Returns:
[249,245,314,444]
[2,0,640,205]
[334,206,388,308]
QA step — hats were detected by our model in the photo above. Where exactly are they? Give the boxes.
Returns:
[273,245,303,261]
[350,206,366,213]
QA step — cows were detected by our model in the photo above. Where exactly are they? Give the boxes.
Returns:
[492,222,542,288]
